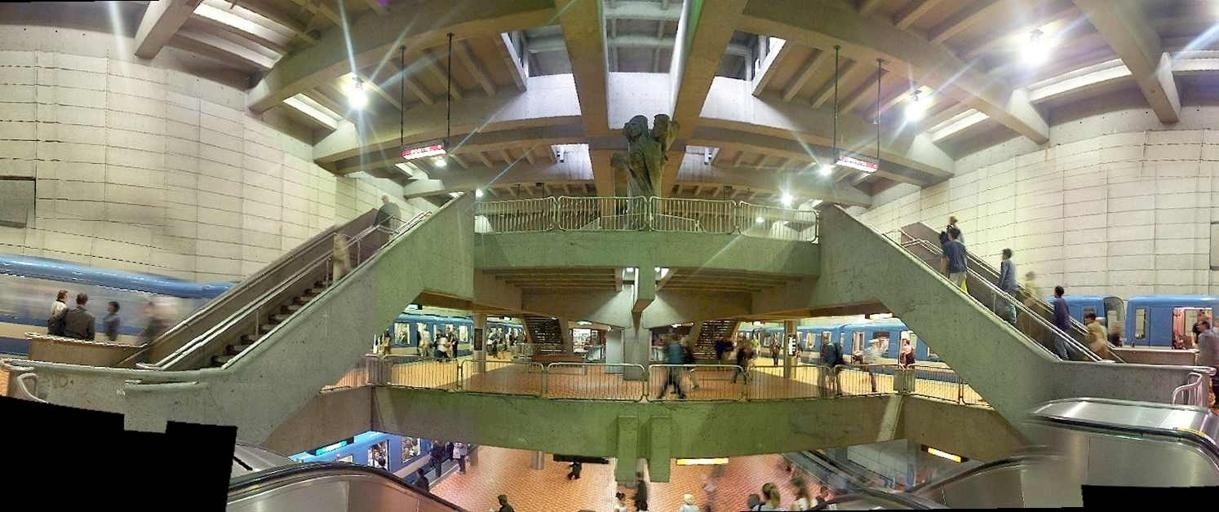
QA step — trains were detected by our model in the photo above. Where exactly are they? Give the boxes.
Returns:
[373,311,525,360]
[1047,297,1219,355]
[0,249,237,369]
[736,321,967,389]
[290,429,453,485]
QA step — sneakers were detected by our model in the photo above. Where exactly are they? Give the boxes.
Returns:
[567,472,580,480]
[456,470,465,475]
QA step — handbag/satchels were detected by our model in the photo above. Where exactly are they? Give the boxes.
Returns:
[452,446,461,459]
[48,313,65,336]
[429,458,434,466]
[938,231,949,243]
[1087,333,1107,352]
[831,342,845,367]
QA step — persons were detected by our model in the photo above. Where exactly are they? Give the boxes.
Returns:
[567,455,582,479]
[373,196,401,245]
[373,323,518,364]
[498,494,514,512]
[368,438,467,492]
[656,330,915,399]
[613,471,829,512]
[51,289,68,317]
[1196,319,1219,409]
[622,114,669,228]
[136,302,163,364]
[1190,309,1210,348]
[332,225,352,282]
[102,302,121,341]
[940,215,1108,362]
[47,292,95,341]
